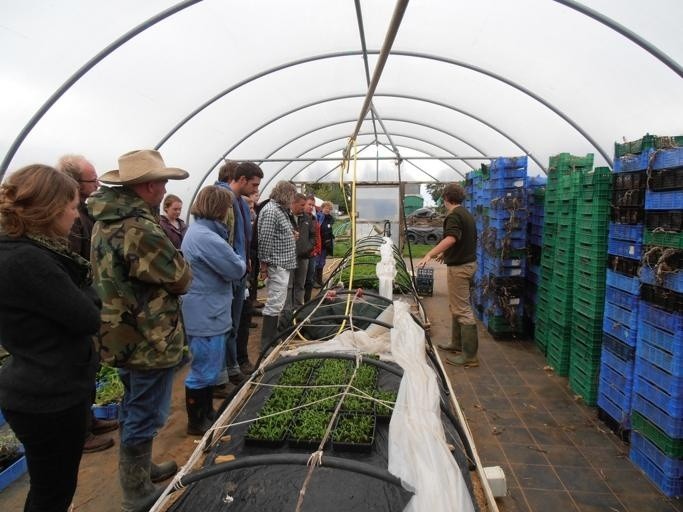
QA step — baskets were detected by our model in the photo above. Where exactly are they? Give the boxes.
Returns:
[598,134,683,500]
[457,134,682,499]
[532,152,616,406]
[462,156,545,344]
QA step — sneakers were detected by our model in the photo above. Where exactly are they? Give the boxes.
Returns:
[213,382,237,398]
[241,360,255,375]
[91,418,119,434]
[230,372,246,385]
[79,433,116,454]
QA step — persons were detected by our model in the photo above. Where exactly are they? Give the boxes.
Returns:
[418,185,484,369]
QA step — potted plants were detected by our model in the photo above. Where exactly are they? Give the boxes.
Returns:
[245,354,397,450]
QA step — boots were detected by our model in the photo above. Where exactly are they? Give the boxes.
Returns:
[119,437,177,510]
[258,314,278,358]
[187,386,218,436]
[438,313,463,353]
[446,322,478,367]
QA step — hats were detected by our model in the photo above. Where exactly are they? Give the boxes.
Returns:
[99,148,189,184]
